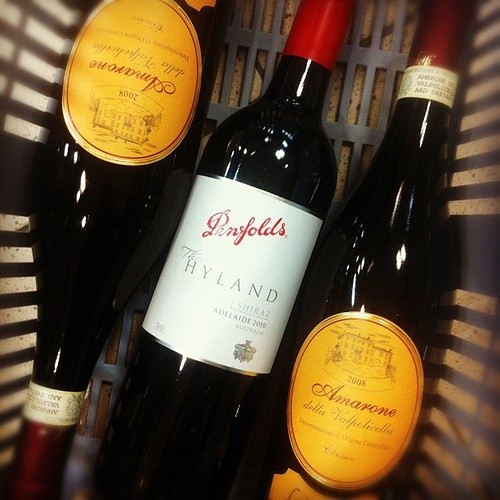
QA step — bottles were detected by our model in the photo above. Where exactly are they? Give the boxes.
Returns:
[1,0,229,499]
[205,0,476,500]
[81,0,365,500]
